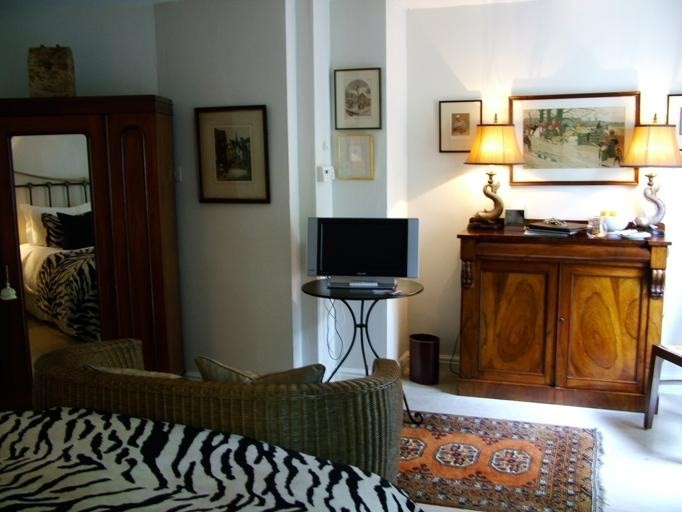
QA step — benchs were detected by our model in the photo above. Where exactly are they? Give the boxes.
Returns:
[34,337,404,490]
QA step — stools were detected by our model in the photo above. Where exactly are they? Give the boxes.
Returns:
[640,342,682,430]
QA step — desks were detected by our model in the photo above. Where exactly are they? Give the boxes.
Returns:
[301,276,426,426]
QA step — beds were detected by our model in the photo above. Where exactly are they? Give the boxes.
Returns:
[14,179,101,339]
[1,405,423,512]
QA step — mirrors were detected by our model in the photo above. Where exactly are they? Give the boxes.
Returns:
[7,133,106,380]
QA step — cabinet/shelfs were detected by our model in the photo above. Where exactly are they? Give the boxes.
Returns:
[1,92,187,409]
[457,217,672,415]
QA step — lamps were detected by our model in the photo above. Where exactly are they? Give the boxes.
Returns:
[464,112,529,231]
[617,111,681,236]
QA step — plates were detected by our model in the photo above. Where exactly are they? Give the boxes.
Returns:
[617,233,651,240]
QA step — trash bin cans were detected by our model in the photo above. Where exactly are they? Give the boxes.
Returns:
[410,334,440,385]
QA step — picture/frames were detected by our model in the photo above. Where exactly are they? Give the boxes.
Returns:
[507,90,641,189]
[190,101,274,206]
[437,99,483,154]
[334,131,376,182]
[332,67,382,131]
[664,92,681,153]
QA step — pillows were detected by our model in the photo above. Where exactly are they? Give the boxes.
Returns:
[20,203,85,244]
[253,362,326,385]
[41,213,63,249]
[28,205,91,247]
[192,353,256,386]
[79,357,182,381]
[60,211,97,251]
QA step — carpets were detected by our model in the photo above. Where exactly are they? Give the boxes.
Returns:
[397,408,609,512]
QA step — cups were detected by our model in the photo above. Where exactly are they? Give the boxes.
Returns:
[599,210,618,233]
[586,217,607,239]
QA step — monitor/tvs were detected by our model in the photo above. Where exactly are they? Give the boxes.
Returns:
[307,216,419,289]
